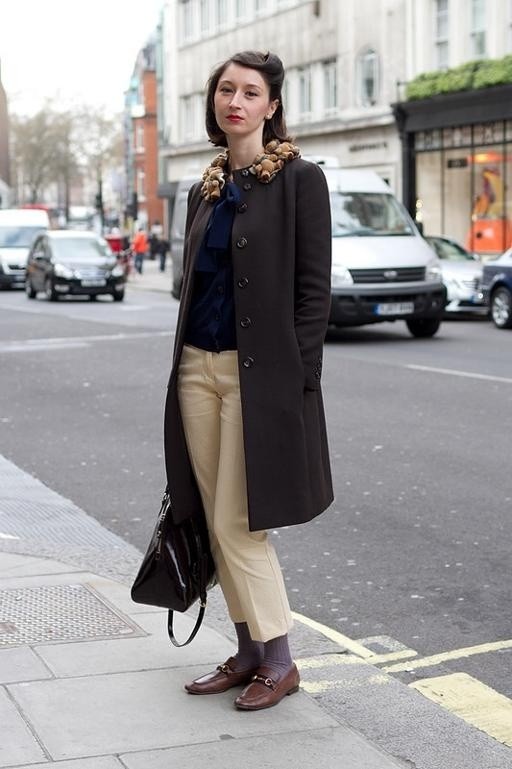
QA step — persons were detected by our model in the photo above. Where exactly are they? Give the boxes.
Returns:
[130,221,169,275]
[164,51,334,711]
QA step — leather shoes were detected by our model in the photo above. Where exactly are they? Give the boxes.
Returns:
[185,655,262,693]
[232,663,301,712]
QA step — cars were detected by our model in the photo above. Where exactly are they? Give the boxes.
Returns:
[24,231,126,304]
[426,235,492,322]
[481,244,512,329]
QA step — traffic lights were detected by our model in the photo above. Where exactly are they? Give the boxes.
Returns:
[126,198,136,220]
[95,194,102,209]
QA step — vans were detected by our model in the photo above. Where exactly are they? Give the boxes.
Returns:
[0,209,53,289]
[168,159,447,338]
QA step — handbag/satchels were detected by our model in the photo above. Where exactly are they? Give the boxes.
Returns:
[129,484,220,609]
